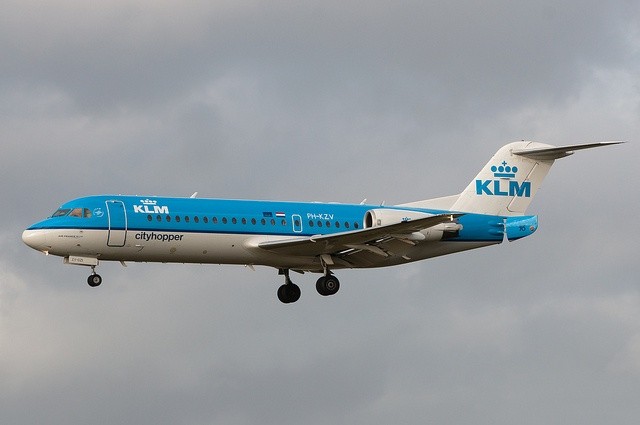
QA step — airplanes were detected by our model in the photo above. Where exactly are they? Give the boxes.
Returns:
[21,141,623,302]
